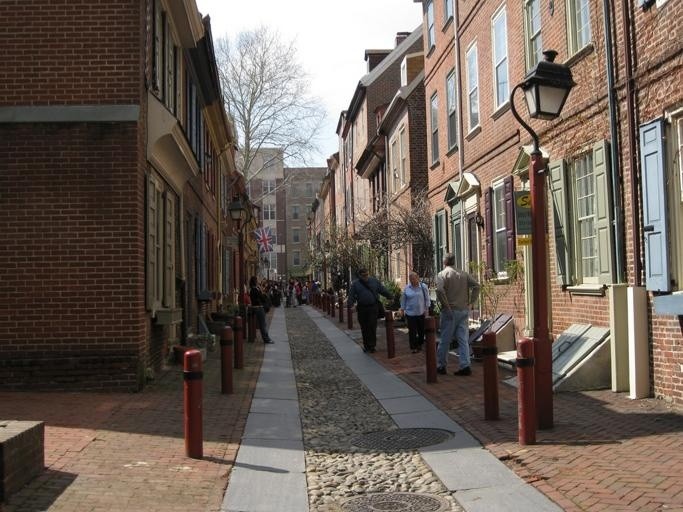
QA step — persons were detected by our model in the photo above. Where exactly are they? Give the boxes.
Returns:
[401,272,431,354]
[348,266,393,352]
[249,275,274,345]
[436,253,480,376]
[261,275,321,308]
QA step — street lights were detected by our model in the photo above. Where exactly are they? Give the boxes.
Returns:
[319,236,331,296]
[226,189,255,342]
[504,44,576,431]
[262,255,270,280]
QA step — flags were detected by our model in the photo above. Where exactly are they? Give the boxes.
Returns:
[254,225,273,254]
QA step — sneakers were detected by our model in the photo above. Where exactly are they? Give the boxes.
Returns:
[437,366,447,375]
[455,367,471,376]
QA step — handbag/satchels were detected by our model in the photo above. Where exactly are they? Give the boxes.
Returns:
[425,308,428,317]
[378,300,385,318]
